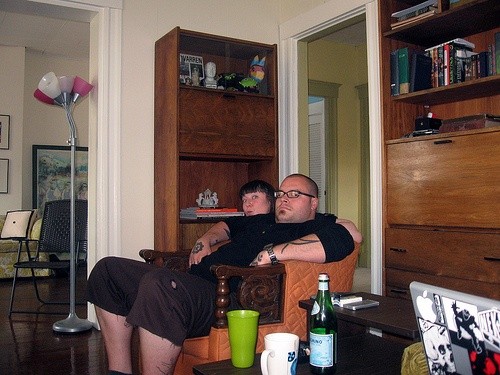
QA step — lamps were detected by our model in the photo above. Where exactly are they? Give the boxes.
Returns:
[34,71,94,335]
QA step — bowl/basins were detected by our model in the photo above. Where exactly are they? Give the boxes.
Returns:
[415,117,442,129]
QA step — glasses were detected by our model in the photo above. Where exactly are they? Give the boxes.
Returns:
[274,190,315,198]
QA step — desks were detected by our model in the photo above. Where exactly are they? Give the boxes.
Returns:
[193,292,421,375]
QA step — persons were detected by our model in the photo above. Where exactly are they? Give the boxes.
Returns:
[83,173,362,375]
[240,180,275,216]
[200,62,217,86]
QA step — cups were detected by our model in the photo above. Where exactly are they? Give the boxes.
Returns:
[226,310,260,368]
[260,332,299,375]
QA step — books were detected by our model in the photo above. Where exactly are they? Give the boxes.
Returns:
[439,114,500,133]
[391,0,466,30]
[179,209,245,219]
[389,31,500,96]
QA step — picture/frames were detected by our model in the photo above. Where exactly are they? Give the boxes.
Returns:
[32,145,88,219]
[0,159,9,194]
[0,115,10,149]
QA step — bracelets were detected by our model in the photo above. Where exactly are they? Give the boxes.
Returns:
[267,246,279,264]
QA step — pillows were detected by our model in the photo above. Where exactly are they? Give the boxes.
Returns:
[27,209,38,240]
[1,210,34,239]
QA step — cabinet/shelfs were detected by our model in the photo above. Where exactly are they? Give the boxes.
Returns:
[154,26,279,266]
[378,0,500,344]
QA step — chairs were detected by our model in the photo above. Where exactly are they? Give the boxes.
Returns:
[8,199,88,317]
[129,240,360,375]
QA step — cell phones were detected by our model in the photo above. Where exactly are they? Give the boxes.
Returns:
[342,299,380,311]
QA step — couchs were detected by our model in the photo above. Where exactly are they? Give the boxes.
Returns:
[0,208,56,278]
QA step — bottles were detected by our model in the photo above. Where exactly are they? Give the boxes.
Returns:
[309,273,337,375]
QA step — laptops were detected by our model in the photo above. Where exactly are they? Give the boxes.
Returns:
[409,281,500,375]
